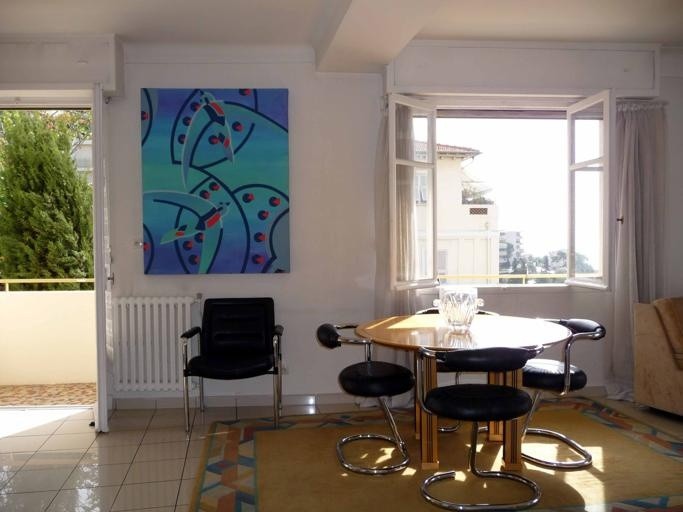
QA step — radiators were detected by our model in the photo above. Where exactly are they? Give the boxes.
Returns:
[111,293,200,401]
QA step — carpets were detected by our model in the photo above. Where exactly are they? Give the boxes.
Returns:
[187,389,682,510]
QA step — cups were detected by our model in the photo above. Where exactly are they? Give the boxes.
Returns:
[438,284,477,335]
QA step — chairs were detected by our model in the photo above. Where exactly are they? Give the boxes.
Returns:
[629,296,682,417]
[180,297,286,433]
